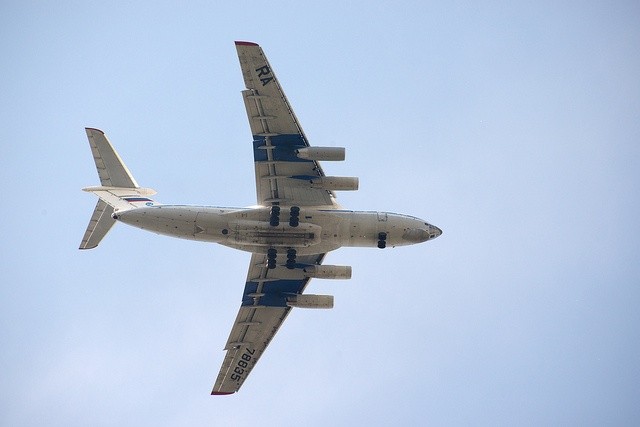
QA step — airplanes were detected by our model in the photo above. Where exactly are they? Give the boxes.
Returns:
[79,41,442,395]
